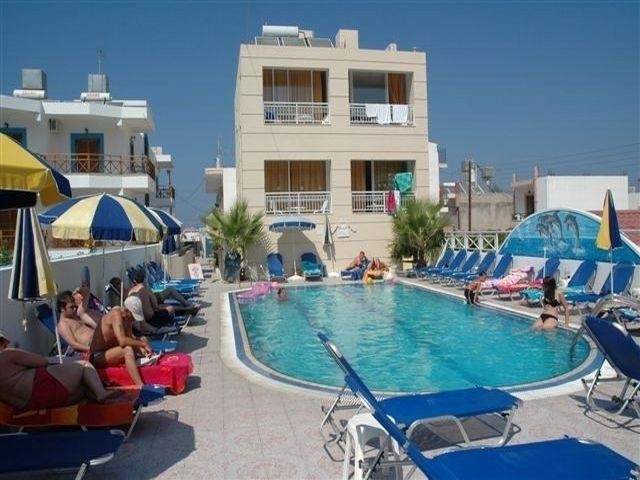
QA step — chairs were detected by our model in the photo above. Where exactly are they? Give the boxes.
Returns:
[414,243,640,418]
[345,375,640,480]
[0,262,202,444]
[266,254,288,284]
[300,252,324,282]
[341,260,370,281]
[316,332,519,463]
[1,429,126,480]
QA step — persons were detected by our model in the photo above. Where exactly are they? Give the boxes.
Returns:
[72,285,152,355]
[55,291,164,400]
[349,251,363,271]
[0,335,125,409]
[464,271,487,308]
[125,272,174,328]
[531,277,569,333]
[278,287,287,300]
[361,258,384,280]
[478,263,533,288]
[109,276,201,316]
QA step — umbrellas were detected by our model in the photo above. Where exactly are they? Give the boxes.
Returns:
[38,190,166,305]
[268,215,315,274]
[8,206,62,364]
[139,203,183,287]
[0,131,74,212]
[595,187,624,293]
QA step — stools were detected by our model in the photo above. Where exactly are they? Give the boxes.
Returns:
[343,414,403,480]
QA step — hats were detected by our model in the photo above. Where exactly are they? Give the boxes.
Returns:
[124,296,144,321]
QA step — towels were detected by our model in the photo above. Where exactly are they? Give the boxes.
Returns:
[376,104,392,125]
[393,105,408,122]
[365,103,377,117]
